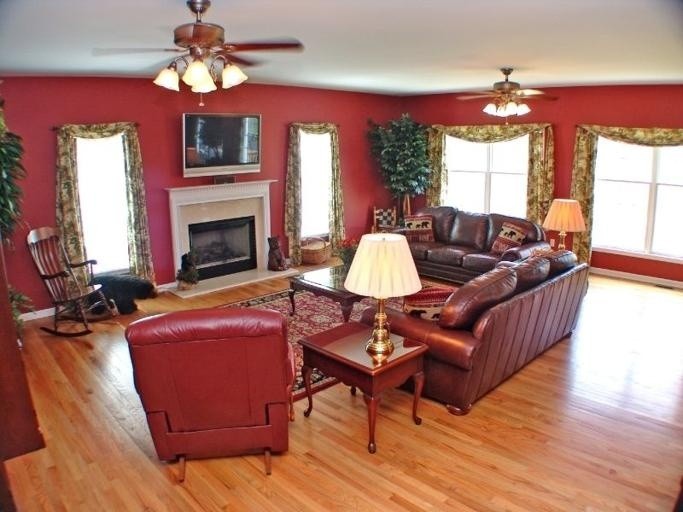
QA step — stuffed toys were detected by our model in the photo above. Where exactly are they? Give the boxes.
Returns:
[267,234,288,271]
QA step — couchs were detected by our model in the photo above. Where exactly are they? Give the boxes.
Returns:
[389,206,545,284]
[358,251,590,418]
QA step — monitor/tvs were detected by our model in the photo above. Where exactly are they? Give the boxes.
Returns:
[181,111,262,184]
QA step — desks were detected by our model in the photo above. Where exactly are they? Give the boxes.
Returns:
[296,322,429,453]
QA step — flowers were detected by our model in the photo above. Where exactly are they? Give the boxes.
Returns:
[329,237,360,270]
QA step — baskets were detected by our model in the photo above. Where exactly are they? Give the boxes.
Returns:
[301,235,332,265]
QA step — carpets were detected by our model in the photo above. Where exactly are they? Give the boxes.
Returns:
[213,279,458,404]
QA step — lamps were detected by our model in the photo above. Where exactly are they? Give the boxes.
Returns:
[481,97,531,118]
[343,235,422,360]
[153,47,250,93]
[542,198,586,250]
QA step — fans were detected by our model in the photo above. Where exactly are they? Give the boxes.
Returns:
[94,0,305,67]
[456,69,560,103]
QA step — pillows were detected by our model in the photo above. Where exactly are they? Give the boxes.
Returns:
[404,206,538,253]
[439,252,579,327]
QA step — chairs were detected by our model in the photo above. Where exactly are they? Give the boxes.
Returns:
[25,226,120,336]
[371,205,395,233]
[124,307,294,481]
